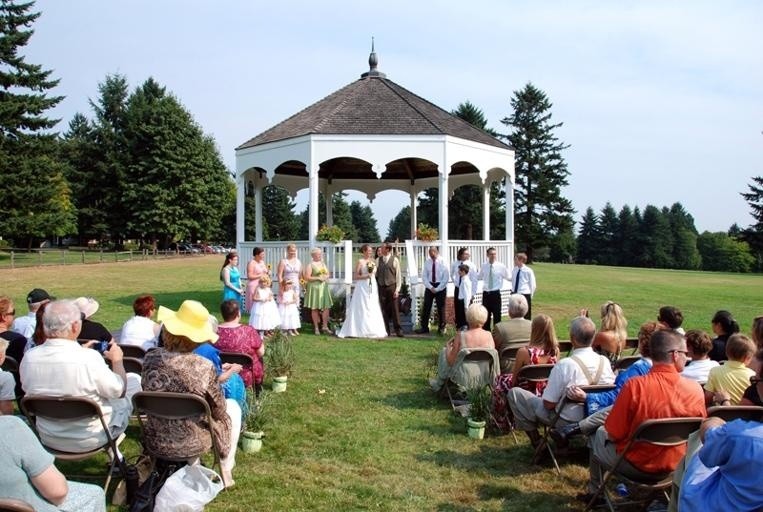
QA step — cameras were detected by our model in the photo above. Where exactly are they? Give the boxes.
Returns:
[93,342,112,355]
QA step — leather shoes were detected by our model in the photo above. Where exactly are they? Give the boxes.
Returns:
[576,492,605,507]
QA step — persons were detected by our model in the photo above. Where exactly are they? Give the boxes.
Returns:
[246,247,269,339]
[703,333,757,406]
[374,246,382,259]
[550,330,707,512]
[414,246,449,335]
[10,287,56,354]
[746,316,763,370]
[278,278,301,336]
[376,242,405,337]
[24,300,49,354]
[510,253,537,320]
[303,247,334,336]
[658,306,687,336]
[0,416,105,512]
[678,329,721,384]
[490,294,533,374]
[212,299,265,387]
[277,244,306,335]
[666,348,763,512]
[19,299,144,454]
[455,265,473,330]
[450,248,477,305]
[335,244,388,339]
[190,343,250,434]
[579,301,628,373]
[140,299,242,488]
[566,321,660,417]
[0,338,29,425]
[428,305,496,398]
[219,254,244,315]
[507,317,616,465]
[75,297,115,352]
[119,296,162,350]
[248,276,282,337]
[708,310,740,362]
[477,247,513,331]
[0,295,27,363]
[490,314,560,435]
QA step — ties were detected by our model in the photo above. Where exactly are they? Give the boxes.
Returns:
[384,257,387,264]
[459,262,463,285]
[489,264,493,289]
[515,269,520,293]
[432,259,435,282]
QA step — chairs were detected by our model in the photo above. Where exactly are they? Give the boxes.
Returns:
[6,320,262,399]
[582,419,704,512]
[500,334,643,370]
[17,397,126,504]
[438,349,494,413]
[499,364,553,446]
[130,388,227,490]
[707,402,762,425]
[530,385,617,475]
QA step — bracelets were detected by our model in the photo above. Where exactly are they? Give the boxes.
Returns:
[721,399,730,406]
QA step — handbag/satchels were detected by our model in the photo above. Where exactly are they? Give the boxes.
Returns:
[130,463,174,512]
[153,457,224,512]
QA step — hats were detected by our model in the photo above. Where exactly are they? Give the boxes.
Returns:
[27,289,56,303]
[157,300,219,344]
[72,297,98,319]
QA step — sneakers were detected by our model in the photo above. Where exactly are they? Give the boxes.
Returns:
[430,379,441,391]
[113,456,126,472]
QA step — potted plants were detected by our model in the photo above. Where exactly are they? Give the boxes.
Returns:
[459,374,495,441]
[260,327,293,391]
[239,390,279,453]
[317,222,345,244]
[413,224,438,242]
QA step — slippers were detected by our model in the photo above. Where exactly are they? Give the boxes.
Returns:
[221,479,235,488]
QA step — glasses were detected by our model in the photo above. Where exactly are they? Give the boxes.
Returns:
[76,312,85,324]
[6,308,15,315]
[750,376,762,384]
[668,349,688,357]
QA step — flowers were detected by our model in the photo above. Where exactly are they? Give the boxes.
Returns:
[366,261,377,289]
[299,278,307,295]
[319,268,327,286]
